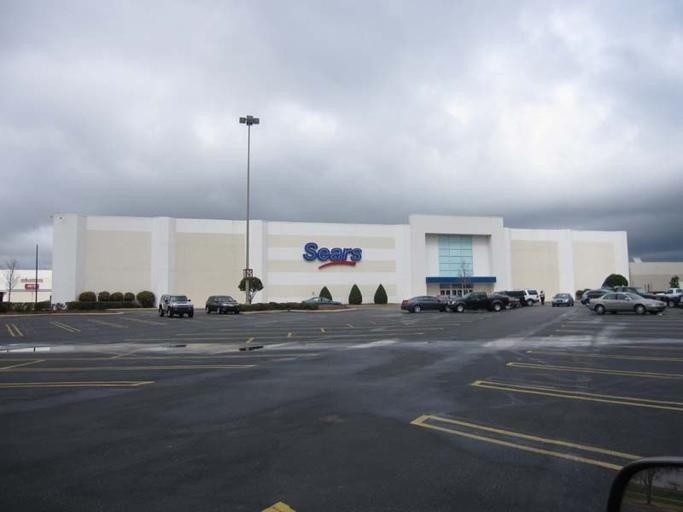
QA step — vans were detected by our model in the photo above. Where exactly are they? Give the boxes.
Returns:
[494,291,539,308]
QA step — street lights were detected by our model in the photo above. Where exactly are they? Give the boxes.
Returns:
[239,116,259,305]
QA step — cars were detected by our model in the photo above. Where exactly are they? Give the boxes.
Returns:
[552,293,574,306]
[206,296,240,314]
[580,286,683,315]
[400,296,455,312]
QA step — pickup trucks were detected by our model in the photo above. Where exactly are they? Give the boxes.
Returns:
[447,291,509,313]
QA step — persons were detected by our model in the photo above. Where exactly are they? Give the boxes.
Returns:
[539,291,545,305]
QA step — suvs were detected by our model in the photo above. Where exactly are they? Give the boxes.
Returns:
[158,294,194,318]
